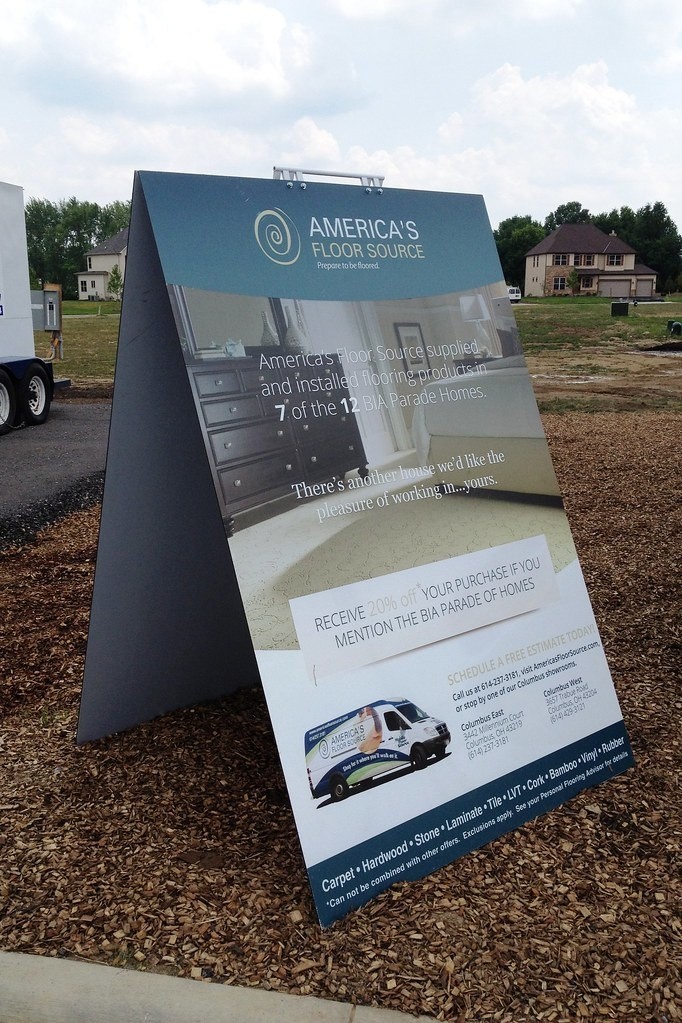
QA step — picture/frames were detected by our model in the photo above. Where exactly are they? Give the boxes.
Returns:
[394,321,432,378]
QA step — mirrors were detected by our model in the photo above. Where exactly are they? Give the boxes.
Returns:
[166,284,288,364]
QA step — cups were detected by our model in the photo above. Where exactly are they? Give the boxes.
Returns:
[462,340,475,359]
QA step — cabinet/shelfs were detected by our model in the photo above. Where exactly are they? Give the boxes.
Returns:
[184,353,370,539]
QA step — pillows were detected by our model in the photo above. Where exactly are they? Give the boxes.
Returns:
[496,327,513,358]
[511,327,523,355]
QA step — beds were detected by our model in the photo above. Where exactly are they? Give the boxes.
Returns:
[410,297,566,512]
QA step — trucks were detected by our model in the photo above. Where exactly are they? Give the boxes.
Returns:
[0,183,64,437]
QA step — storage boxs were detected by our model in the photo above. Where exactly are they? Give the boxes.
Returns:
[611,302,629,317]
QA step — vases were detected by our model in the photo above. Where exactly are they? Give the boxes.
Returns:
[282,305,309,355]
[260,310,280,347]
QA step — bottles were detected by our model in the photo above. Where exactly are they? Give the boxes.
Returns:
[634,301,637,306]
[261,307,280,346]
[284,306,309,355]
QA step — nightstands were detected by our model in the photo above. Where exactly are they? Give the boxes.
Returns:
[452,358,500,376]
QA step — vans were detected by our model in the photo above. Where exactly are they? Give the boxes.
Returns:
[507,287,521,303]
[304,695,451,802]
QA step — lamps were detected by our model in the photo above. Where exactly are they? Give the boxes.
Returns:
[458,294,494,357]
[596,282,598,284]
[654,282,655,284]
[632,282,634,284]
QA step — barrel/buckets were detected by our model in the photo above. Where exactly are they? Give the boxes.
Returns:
[88,295,99,301]
[668,321,681,335]
[611,303,628,315]
